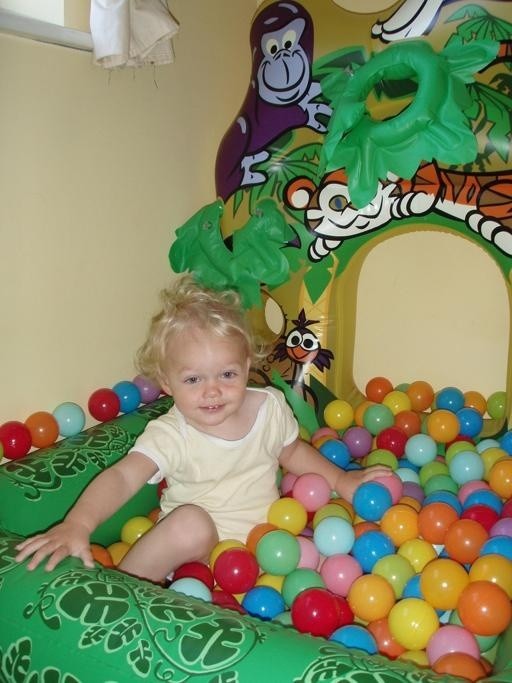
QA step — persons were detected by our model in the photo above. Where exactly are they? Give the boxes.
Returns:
[13,270,393,588]
